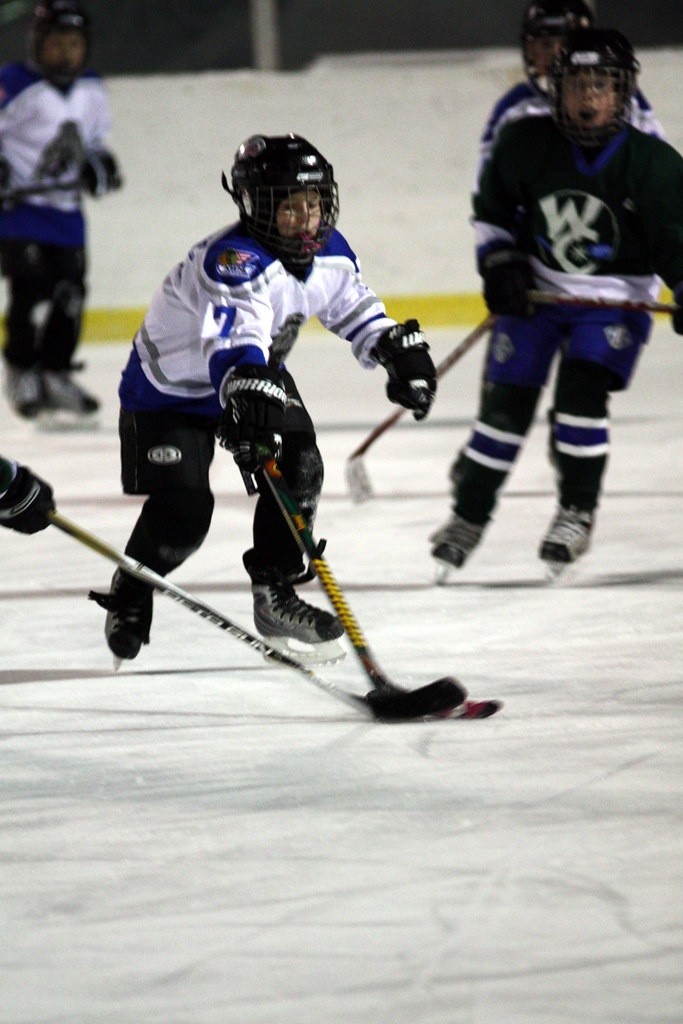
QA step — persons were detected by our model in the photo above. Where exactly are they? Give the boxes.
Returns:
[89,132,440,661]
[0,453,55,537]
[1,0,126,419]
[428,0,682,570]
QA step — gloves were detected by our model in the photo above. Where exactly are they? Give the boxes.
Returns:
[1,457,57,536]
[370,317,437,423]
[219,366,290,474]
[474,238,542,320]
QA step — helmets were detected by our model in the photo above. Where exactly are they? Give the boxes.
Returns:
[19,0,90,98]
[522,0,595,99]
[552,28,637,149]
[222,131,340,275]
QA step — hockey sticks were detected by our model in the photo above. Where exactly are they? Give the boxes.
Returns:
[45,510,467,722]
[256,458,503,721]
[341,316,509,502]
[530,289,683,314]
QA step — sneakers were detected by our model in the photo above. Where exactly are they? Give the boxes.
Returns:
[3,356,101,431]
[86,564,153,677]
[428,488,497,585]
[251,565,346,668]
[536,478,602,578]
